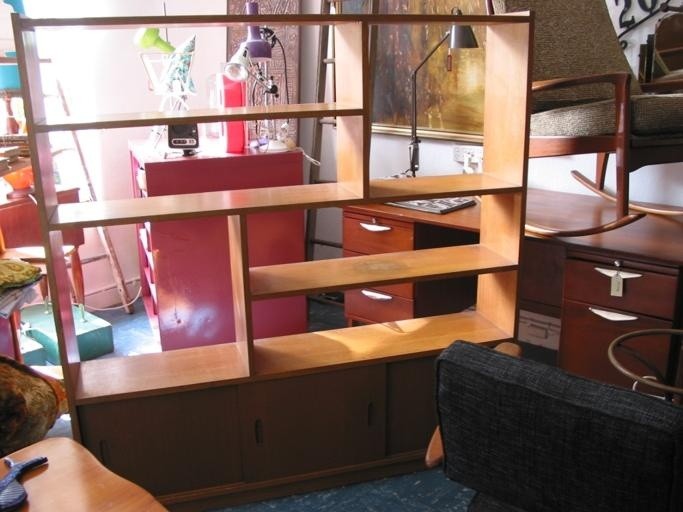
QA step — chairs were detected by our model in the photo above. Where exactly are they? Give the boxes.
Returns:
[485,1,683,237]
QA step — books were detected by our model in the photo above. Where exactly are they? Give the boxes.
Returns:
[383,193,478,215]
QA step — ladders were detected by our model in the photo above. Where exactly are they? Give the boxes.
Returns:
[0,56,132,315]
[305,1,380,307]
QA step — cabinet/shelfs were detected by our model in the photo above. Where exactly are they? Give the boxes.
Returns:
[127,143,310,354]
[10,9,539,509]
[561,256,681,399]
[342,208,413,328]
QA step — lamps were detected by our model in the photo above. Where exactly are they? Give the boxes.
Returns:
[225,41,277,148]
[391,7,480,181]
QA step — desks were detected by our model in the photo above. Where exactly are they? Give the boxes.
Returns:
[2,435,168,510]
[1,175,86,311]
